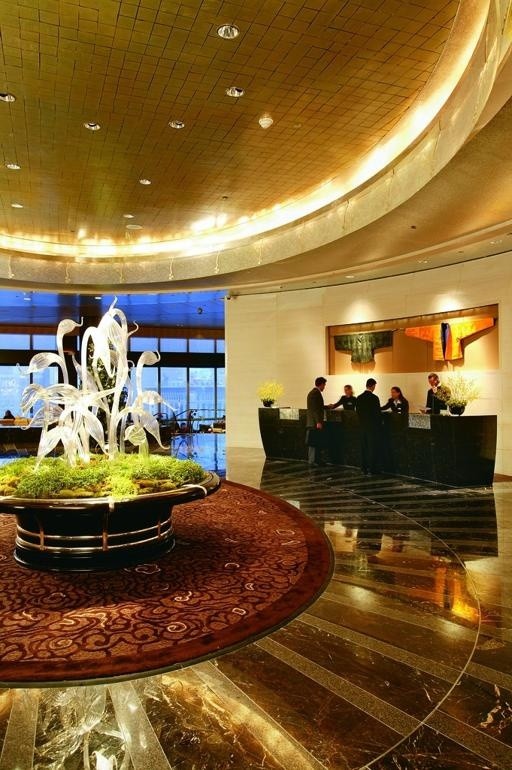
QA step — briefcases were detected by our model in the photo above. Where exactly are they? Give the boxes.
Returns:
[304,428,330,447]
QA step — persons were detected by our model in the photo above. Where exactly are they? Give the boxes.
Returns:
[380,386,408,416]
[298,468,325,532]
[356,474,384,565]
[331,385,357,411]
[418,373,452,415]
[4,409,17,419]
[355,378,384,474]
[306,376,333,468]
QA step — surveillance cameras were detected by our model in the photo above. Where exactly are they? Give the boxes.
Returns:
[226,294,232,301]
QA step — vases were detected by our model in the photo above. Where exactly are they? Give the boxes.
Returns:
[447,402,466,415]
[262,399,273,407]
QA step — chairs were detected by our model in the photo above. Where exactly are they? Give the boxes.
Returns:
[0,416,33,426]
